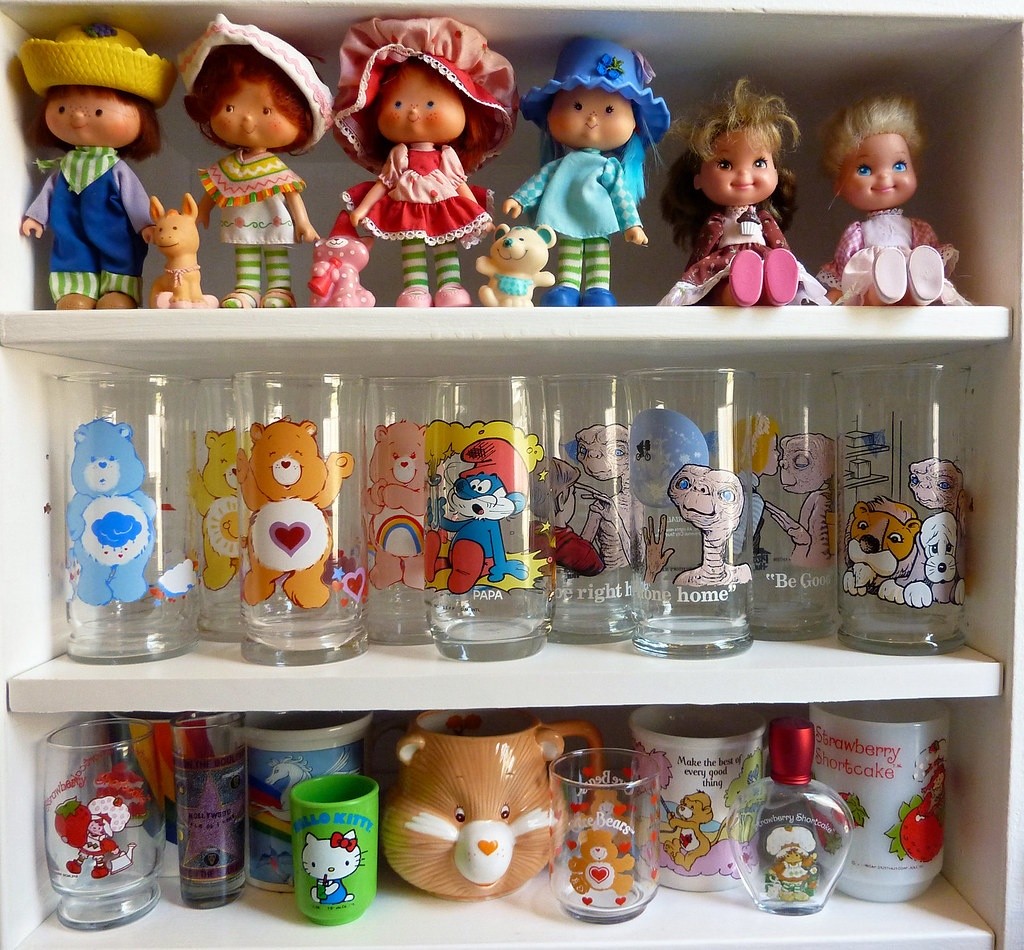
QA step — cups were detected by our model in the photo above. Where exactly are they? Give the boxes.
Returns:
[537,374,636,645]
[429,377,549,664]
[172,712,248,909]
[830,363,978,651]
[361,379,433,645]
[290,776,378,926]
[631,708,767,892]
[42,718,161,931]
[188,378,247,643]
[52,373,201,665]
[620,368,754,657]
[109,712,182,876]
[549,748,661,923]
[232,711,372,892]
[380,710,602,899]
[809,703,950,904]
[232,372,369,666]
[745,374,841,642]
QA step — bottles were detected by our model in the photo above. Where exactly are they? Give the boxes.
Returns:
[727,722,857,915]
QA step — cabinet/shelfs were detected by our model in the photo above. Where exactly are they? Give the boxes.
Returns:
[1,0,1023,948]
